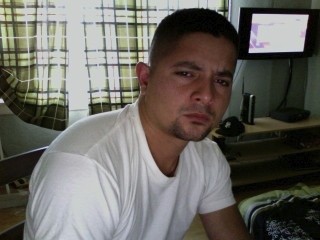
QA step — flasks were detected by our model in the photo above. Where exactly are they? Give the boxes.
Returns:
[242,93,256,125]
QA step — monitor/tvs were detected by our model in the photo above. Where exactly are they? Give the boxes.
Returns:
[238,6,319,60]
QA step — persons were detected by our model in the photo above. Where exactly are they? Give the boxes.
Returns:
[23,7,252,239]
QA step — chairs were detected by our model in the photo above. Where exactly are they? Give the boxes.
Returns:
[0,147,49,239]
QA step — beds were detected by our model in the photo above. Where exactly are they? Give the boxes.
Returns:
[236,182,320,240]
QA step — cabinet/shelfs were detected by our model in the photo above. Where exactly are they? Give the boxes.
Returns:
[209,113,320,185]
